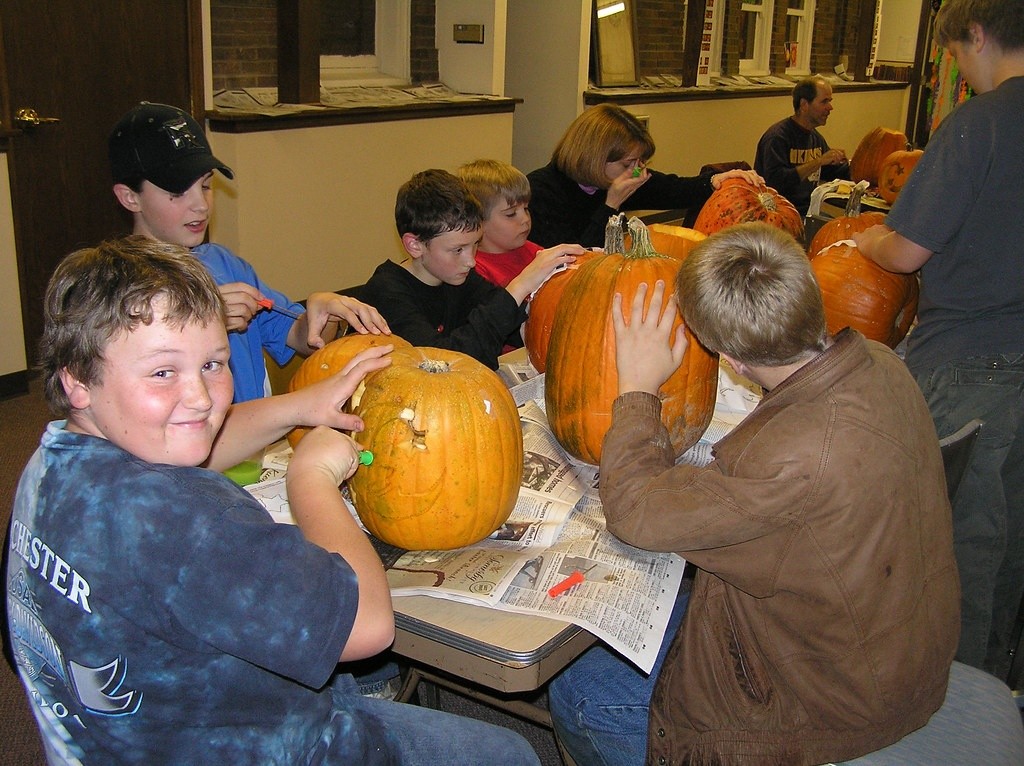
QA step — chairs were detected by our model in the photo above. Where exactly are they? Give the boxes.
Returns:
[832,659,1024,766]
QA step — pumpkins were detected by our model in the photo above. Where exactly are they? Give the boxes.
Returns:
[286,332,411,452]
[543,217,719,468]
[523,212,625,373]
[808,185,890,258]
[692,177,803,241]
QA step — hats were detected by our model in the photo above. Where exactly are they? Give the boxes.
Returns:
[108,101,235,193]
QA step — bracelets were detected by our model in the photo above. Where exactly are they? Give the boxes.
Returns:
[710,173,717,182]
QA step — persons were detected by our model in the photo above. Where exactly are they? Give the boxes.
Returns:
[5,235,540,766]
[104,100,394,406]
[852,1,1024,691]
[753,76,851,216]
[357,159,587,377]
[525,102,765,248]
[547,220,961,764]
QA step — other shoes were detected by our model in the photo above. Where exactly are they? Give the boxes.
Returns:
[362,681,399,702]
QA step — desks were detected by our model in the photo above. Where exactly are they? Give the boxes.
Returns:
[248,349,765,766]
